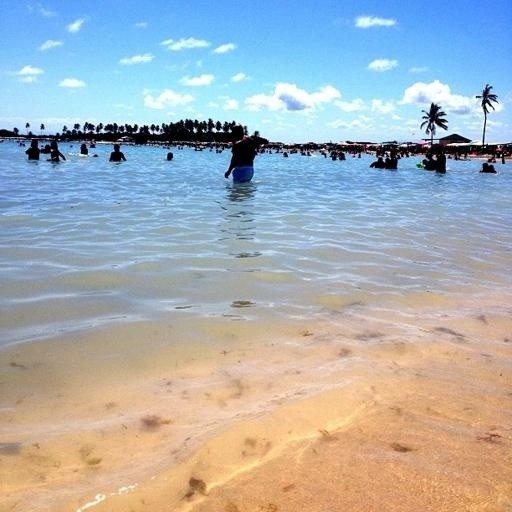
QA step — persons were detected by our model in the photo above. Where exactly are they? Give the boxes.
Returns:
[0,136,233,164]
[224,125,257,186]
[255,140,512,175]
